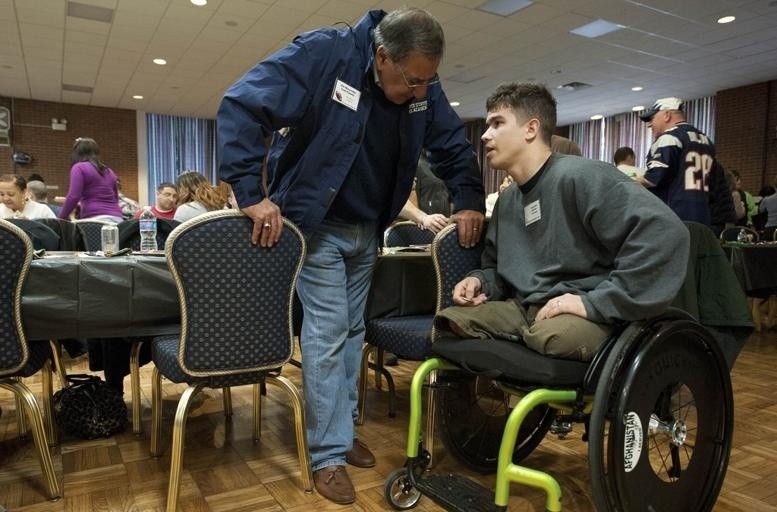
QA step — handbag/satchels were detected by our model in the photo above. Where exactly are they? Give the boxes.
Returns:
[53,374,128,440]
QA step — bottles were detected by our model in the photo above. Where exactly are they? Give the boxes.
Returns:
[736,228,746,243]
[139,206,157,251]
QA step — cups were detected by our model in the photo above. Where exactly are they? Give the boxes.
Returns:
[99,222,120,253]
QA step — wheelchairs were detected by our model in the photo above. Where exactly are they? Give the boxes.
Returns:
[384,226,735,512]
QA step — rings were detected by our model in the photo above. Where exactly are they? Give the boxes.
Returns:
[264,222,272,230]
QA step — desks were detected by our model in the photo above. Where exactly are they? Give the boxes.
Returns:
[17,248,439,343]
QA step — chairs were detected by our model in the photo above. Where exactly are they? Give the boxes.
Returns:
[0,212,186,461]
[357,220,489,472]
[149,209,314,512]
[717,224,776,337]
[1,218,63,503]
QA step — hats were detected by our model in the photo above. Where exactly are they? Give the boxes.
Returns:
[639,97,682,122]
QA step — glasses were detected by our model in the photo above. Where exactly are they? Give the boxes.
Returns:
[398,64,440,88]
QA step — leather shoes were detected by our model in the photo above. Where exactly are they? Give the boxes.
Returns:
[346,439,375,468]
[313,466,357,504]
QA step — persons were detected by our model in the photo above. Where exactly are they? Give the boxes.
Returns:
[216,5,486,504]
[639,97,714,227]
[710,159,777,242]
[614,147,647,180]
[399,150,514,235]
[550,129,582,156]
[0,136,240,222]
